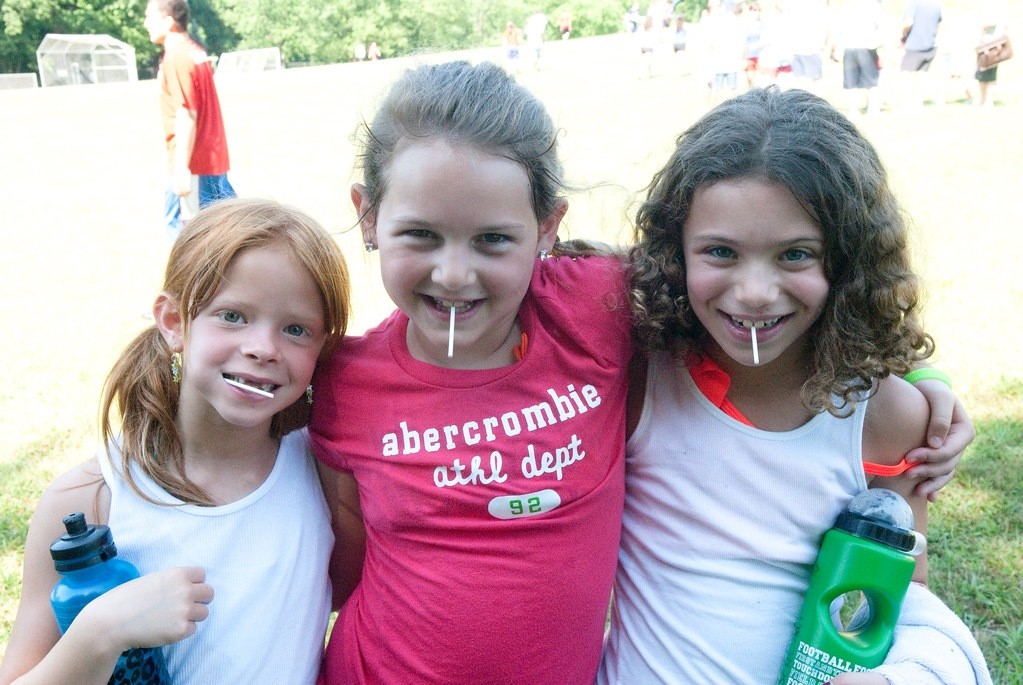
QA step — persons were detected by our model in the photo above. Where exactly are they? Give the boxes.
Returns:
[143,0,237,228]
[594,85,932,685]
[3,196,350,685]
[727,0,1023,110]
[309,61,976,685]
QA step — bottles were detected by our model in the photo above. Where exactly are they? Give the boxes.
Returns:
[773,483,921,684]
[48,512,171,684]
